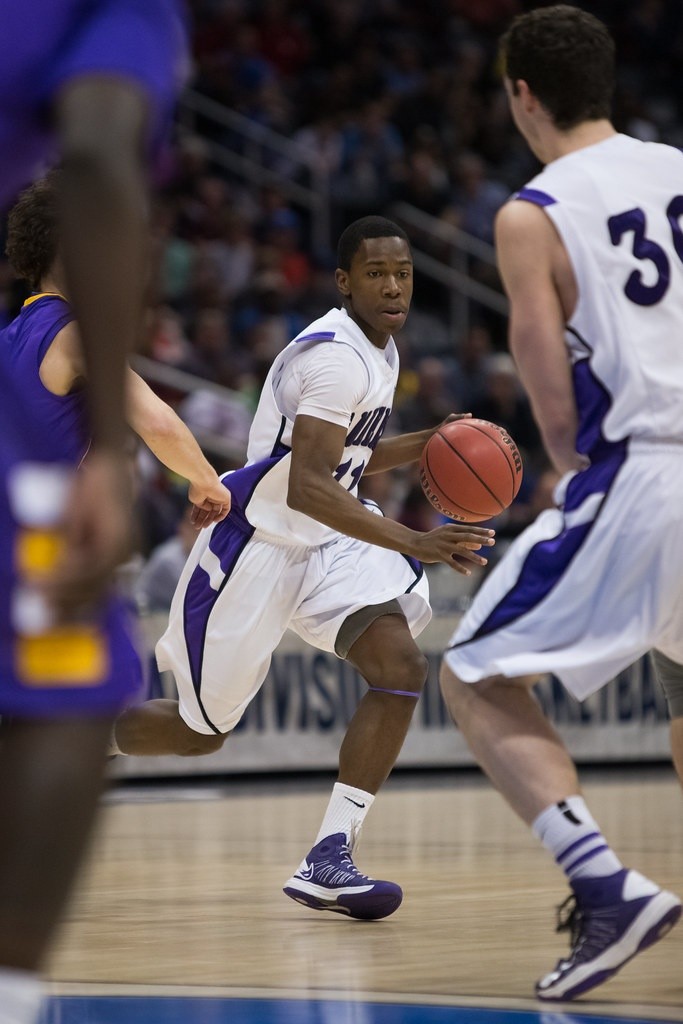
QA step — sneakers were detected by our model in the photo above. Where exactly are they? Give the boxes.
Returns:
[282,819,403,921]
[535,869,682,1001]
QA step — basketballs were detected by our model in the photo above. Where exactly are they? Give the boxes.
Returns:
[420,418,522,522]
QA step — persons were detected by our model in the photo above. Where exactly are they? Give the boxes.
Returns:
[107,218,496,919]
[439,3,681,995]
[127,0,682,737]
[1,0,231,1024]
